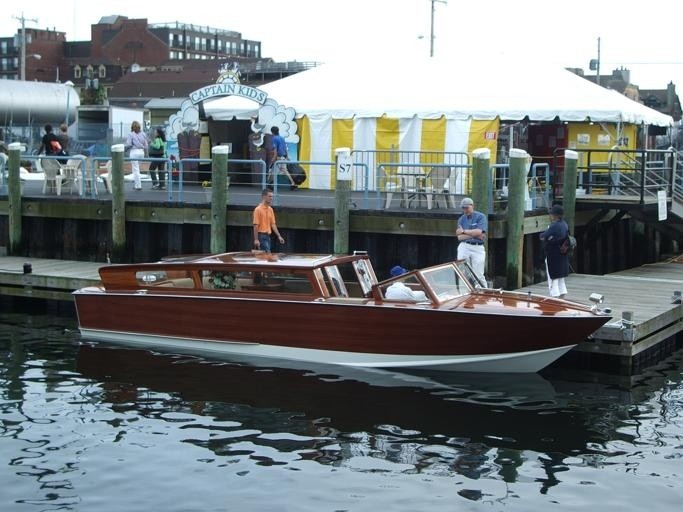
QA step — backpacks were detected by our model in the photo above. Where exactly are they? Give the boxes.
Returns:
[49,140,62,153]
[560,236,577,256]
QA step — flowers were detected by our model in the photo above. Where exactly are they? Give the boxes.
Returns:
[208,272,238,290]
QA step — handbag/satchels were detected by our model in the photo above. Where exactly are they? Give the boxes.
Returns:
[148,145,164,157]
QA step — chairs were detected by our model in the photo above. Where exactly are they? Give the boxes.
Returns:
[41,154,112,195]
[374,162,460,209]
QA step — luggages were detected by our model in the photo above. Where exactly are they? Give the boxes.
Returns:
[287,163,307,183]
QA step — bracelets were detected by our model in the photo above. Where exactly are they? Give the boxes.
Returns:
[462,228,464,233]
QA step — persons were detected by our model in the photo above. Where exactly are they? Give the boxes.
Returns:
[543,204,569,299]
[385,266,419,299]
[456,198,487,288]
[265,127,297,191]
[38,122,70,184]
[125,121,148,191]
[148,128,166,190]
[252,188,285,253]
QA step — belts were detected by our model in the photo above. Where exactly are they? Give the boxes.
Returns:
[466,242,484,245]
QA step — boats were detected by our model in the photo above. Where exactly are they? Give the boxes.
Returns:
[72,250,612,374]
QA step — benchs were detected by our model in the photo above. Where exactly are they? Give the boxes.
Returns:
[154,277,254,291]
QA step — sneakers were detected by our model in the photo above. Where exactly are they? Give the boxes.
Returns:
[290,186,298,191]
[133,187,142,191]
[267,185,274,190]
[153,183,167,190]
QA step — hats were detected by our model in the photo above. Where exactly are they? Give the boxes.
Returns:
[460,198,473,207]
[548,204,564,216]
[390,265,406,276]
